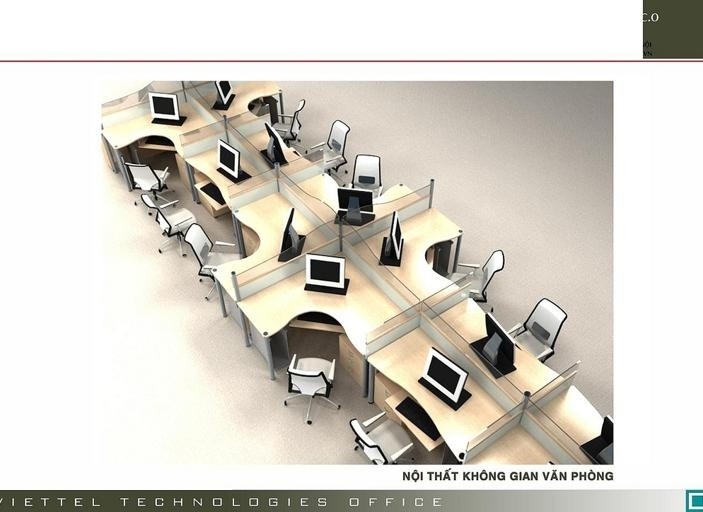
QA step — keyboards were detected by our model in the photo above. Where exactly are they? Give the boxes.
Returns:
[394,396,441,441]
[200,182,226,205]
[296,312,341,326]
[143,136,174,148]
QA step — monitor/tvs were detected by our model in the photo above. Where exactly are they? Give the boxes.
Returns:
[259,121,288,169]
[378,210,405,267]
[482,311,517,370]
[277,207,306,261]
[148,92,187,126]
[215,136,253,185]
[597,414,613,462]
[211,81,236,110]
[422,345,467,406]
[333,188,376,228]
[304,252,350,296]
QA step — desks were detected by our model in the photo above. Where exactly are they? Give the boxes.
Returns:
[101,81,284,192]
[463,368,613,464]
